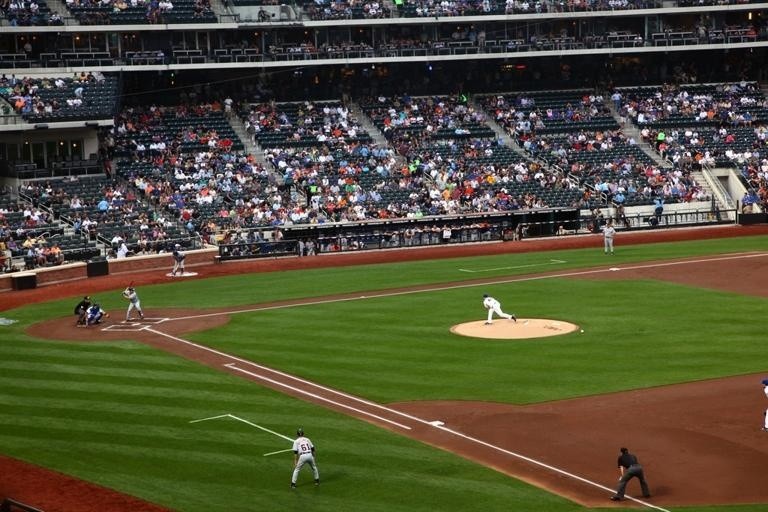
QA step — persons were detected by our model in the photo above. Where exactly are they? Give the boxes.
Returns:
[85,300,109,327]
[761,380,768,431]
[481,291,517,326]
[120,285,145,325]
[73,294,90,326]
[610,444,650,502]
[288,427,320,488]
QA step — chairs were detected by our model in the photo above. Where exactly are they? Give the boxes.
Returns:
[10,62,768,253]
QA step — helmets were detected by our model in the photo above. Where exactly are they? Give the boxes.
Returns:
[93,304,99,311]
[297,428,303,435]
[84,296,90,303]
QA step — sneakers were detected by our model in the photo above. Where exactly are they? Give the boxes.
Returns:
[290,483,296,489]
[77,320,101,328]
[314,479,320,486]
[610,496,619,500]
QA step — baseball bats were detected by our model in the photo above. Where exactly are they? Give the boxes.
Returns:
[127,281,134,291]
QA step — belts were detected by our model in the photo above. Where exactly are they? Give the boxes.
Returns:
[299,452,309,455]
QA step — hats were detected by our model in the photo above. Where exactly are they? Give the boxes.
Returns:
[620,448,628,453]
